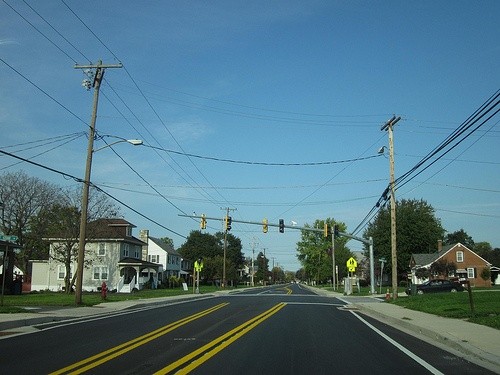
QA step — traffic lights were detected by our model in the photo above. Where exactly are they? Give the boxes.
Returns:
[279,220,285,234]
[333,224,339,239]
[225,215,231,231]
[323,223,332,238]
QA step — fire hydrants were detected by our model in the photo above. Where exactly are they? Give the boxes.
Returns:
[102,281,108,300]
[385,292,390,301]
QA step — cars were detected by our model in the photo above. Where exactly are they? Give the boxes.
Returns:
[405,279,464,295]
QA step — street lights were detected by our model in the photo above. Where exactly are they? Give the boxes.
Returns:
[378,143,399,300]
[75,140,144,306]
